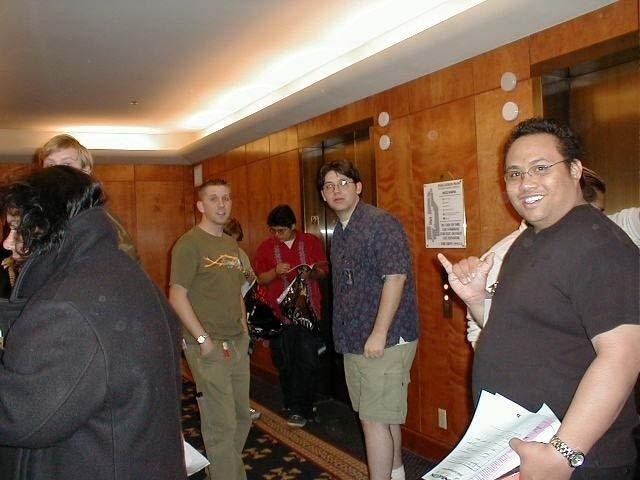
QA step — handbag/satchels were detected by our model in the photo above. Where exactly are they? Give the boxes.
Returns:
[270,325,320,377]
[277,277,319,331]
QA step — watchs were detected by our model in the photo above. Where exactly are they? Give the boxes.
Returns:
[196,334,210,344]
[549,436,585,467]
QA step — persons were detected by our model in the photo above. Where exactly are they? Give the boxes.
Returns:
[168,179,260,479]
[465,167,640,351]
[0,133,186,479]
[221,217,263,421]
[438,116,640,480]
[250,204,330,427]
[315,159,421,479]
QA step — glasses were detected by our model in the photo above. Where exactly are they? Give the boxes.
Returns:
[322,179,355,193]
[503,160,571,186]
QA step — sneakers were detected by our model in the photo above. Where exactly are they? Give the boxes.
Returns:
[287,415,307,427]
